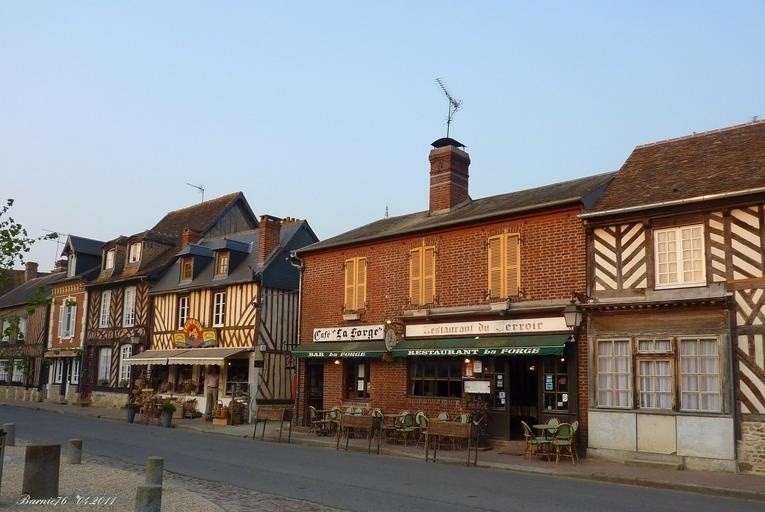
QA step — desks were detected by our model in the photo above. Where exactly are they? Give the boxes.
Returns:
[423,419,477,469]
[335,414,381,456]
[253,407,293,443]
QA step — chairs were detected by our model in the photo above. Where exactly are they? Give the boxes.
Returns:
[305,404,489,452]
[520,417,580,466]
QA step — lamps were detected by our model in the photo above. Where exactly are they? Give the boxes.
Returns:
[561,291,600,343]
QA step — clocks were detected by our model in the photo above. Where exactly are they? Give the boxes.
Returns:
[384,328,398,352]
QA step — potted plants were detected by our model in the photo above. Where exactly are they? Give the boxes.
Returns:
[120,380,129,387]
[127,402,137,424]
[159,404,177,428]
